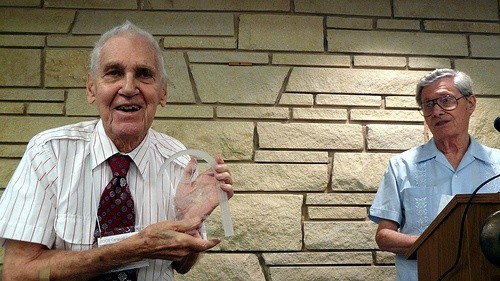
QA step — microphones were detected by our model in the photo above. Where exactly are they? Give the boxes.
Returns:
[494,116,500,132]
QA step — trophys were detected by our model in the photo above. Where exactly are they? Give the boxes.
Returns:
[156,148,233,242]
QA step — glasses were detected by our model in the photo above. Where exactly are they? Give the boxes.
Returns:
[418,93,468,117]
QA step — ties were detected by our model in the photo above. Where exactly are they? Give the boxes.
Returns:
[89,153,137,281]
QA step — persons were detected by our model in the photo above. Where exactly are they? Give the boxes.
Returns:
[0,21,234,281]
[366,69,500,281]
